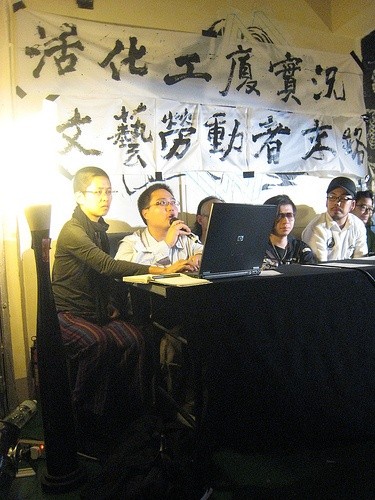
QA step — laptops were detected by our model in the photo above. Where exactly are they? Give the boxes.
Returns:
[182,201,279,280]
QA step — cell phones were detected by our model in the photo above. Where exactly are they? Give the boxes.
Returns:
[152,273,180,279]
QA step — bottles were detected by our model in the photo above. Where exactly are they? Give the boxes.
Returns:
[31,443,46,459]
[0,399,37,435]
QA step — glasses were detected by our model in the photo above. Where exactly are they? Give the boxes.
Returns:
[327,195,352,202]
[360,205,373,212]
[84,190,118,197]
[279,212,296,220]
[147,199,180,209]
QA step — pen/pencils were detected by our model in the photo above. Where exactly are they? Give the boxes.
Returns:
[152,274,180,279]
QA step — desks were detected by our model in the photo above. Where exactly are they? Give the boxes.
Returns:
[120,250,375,499]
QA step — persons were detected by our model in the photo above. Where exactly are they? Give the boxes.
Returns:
[112,182,232,446]
[198,175,374,462]
[54,163,200,465]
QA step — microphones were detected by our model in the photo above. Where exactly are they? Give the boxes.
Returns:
[169,217,201,243]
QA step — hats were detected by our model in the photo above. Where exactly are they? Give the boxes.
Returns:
[326,177,357,198]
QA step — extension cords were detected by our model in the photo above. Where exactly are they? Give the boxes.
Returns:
[18,439,45,459]
